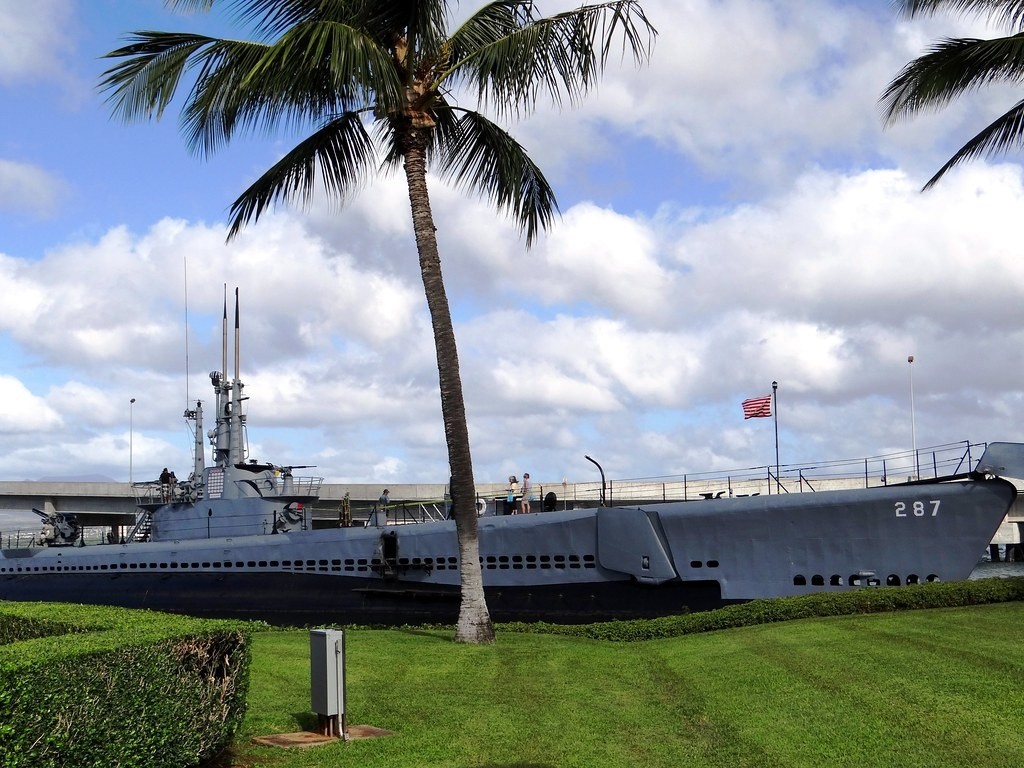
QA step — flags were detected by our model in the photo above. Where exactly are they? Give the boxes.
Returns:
[741,395,772,419]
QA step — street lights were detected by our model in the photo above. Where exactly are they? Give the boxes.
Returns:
[772,381,779,494]
[130,398,136,481]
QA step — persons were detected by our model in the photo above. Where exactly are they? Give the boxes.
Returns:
[159,468,178,504]
[379,488,390,526]
[506,473,531,515]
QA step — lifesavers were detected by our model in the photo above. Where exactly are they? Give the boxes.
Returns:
[476,497,487,516]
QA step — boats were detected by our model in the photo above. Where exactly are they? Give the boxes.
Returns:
[0,258,1018,624]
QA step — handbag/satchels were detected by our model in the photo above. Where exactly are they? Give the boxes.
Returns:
[507,490,514,502]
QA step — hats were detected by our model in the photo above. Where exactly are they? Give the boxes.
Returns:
[383,489,389,493]
